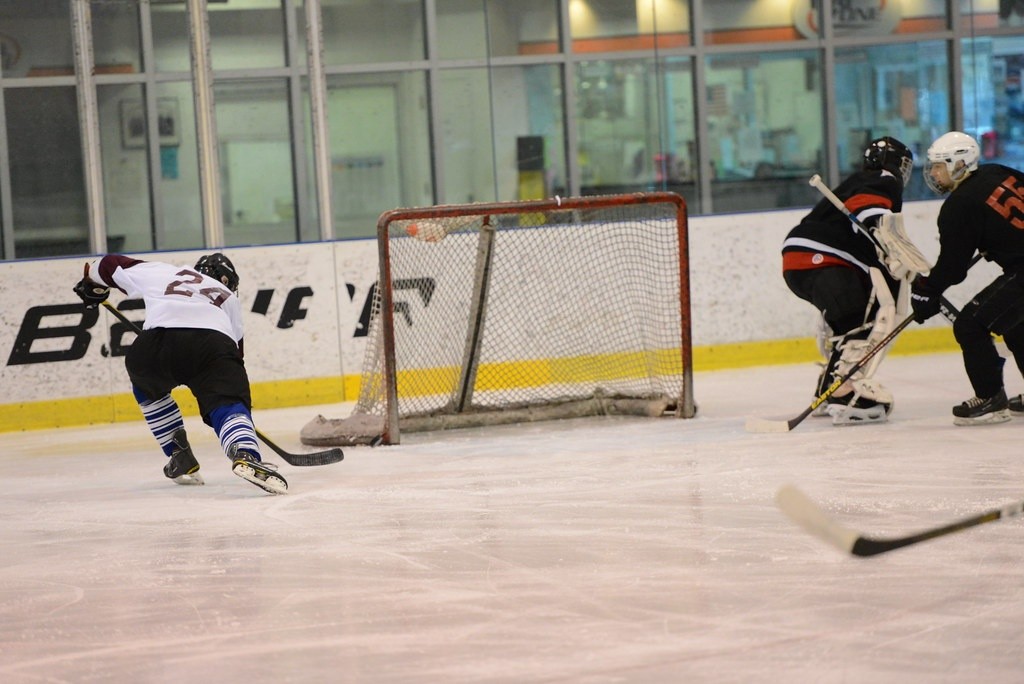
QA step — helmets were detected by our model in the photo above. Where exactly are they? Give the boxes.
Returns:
[864,137,913,178]
[194,252,239,292]
[927,132,980,177]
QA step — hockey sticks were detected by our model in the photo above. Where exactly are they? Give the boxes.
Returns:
[772,483,1024,559]
[741,253,984,435]
[101,298,345,468]
[807,174,962,326]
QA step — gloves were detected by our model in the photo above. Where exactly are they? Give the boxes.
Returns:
[977,246,993,263]
[76,276,111,310]
[910,276,940,324]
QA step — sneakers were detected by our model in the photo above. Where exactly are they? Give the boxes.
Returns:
[952,383,1012,426]
[812,395,830,417]
[1008,393,1024,411]
[163,427,206,487]
[827,392,887,426]
[232,451,287,494]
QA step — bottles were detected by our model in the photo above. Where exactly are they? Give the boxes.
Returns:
[406,222,443,242]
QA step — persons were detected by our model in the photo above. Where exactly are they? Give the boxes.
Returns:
[911,132,1024,427]
[781,136,929,425]
[633,128,875,213]
[98,253,288,496]
